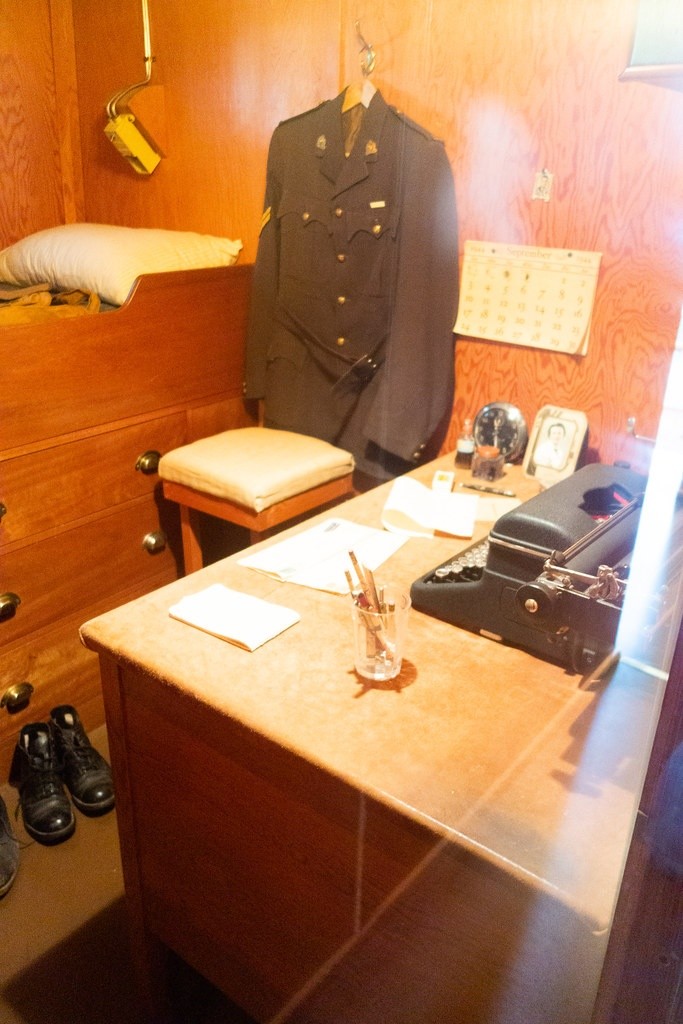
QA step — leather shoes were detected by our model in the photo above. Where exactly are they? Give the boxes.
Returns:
[17,705,116,841]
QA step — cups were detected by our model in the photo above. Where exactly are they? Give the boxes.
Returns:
[352,587,412,682]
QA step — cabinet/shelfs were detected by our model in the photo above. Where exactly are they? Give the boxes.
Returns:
[1,397,257,782]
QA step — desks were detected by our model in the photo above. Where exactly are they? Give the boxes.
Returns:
[77,444,682,1024]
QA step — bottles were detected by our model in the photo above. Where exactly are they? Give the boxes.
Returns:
[454,418,475,470]
[471,445,506,482]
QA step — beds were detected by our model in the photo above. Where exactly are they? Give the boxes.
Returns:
[0,263,257,454]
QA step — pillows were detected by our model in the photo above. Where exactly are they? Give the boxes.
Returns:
[0,223,243,307]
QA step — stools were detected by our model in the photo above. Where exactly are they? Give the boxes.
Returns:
[158,427,354,575]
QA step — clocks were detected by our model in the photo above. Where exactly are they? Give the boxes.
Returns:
[470,403,525,458]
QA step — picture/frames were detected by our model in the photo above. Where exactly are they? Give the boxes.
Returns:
[523,404,589,489]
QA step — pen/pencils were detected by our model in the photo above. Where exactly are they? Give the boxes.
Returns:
[460,482,516,497]
[345,551,395,664]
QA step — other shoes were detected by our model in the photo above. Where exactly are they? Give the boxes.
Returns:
[0,796,20,896]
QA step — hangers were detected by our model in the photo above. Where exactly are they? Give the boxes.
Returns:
[343,45,378,113]
